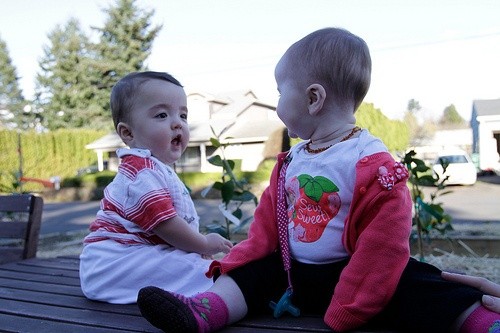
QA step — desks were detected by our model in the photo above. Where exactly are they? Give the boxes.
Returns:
[0,255,330,333]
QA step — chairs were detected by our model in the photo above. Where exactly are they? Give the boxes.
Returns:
[0,195,44,258]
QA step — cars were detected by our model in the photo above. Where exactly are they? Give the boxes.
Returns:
[431,152,477,187]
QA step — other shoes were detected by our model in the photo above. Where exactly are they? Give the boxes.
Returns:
[137,286,197,333]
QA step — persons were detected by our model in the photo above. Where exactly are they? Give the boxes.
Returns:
[79,70,233,303]
[441,271,500,313]
[137,27,500,333]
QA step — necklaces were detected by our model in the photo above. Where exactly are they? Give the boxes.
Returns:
[304,126,361,152]
